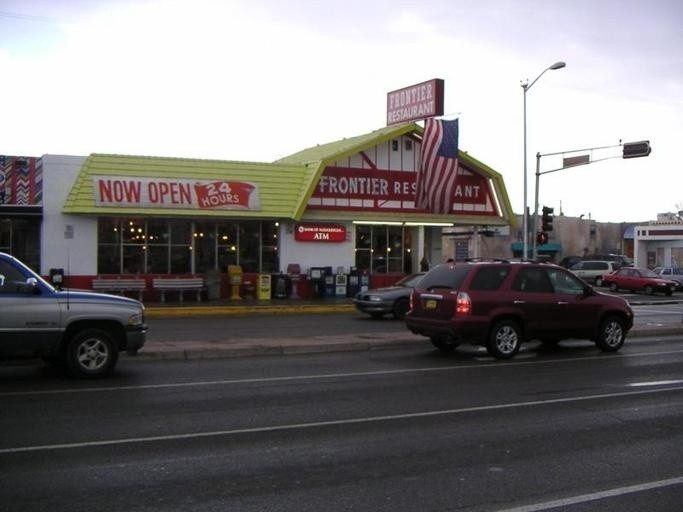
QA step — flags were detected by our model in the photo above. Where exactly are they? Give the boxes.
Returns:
[414,118,460,214]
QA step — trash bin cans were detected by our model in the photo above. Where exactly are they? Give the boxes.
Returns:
[49,268,63,289]
[244,271,287,300]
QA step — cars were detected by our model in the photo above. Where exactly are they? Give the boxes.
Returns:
[568,253,682,298]
[357,268,432,321]
[0,251,150,384]
[410,258,632,362]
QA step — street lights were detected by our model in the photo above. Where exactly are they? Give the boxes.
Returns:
[520,61,566,262]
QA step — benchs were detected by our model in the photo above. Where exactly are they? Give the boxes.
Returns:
[92,279,148,302]
[152,278,205,303]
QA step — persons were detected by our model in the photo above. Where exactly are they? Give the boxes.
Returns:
[418,256,430,272]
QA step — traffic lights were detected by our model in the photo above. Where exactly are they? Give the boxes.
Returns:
[542,207,553,232]
[536,233,549,245]
